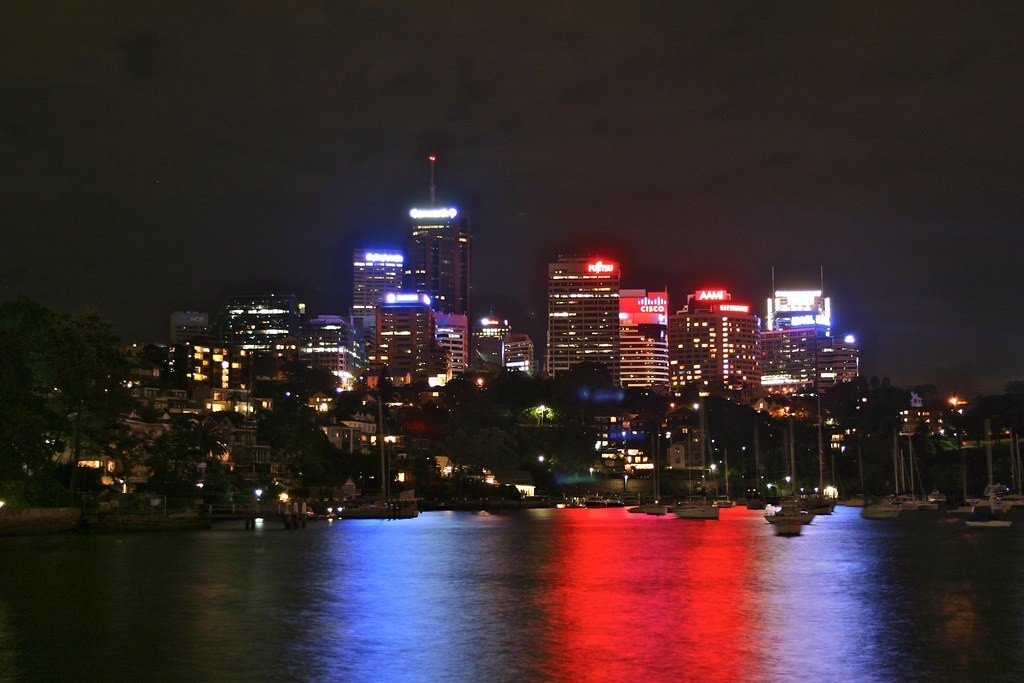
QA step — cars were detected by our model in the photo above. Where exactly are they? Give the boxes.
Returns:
[32,459,128,497]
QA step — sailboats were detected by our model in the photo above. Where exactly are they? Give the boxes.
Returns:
[627,394,1024,537]
[338,396,420,522]
